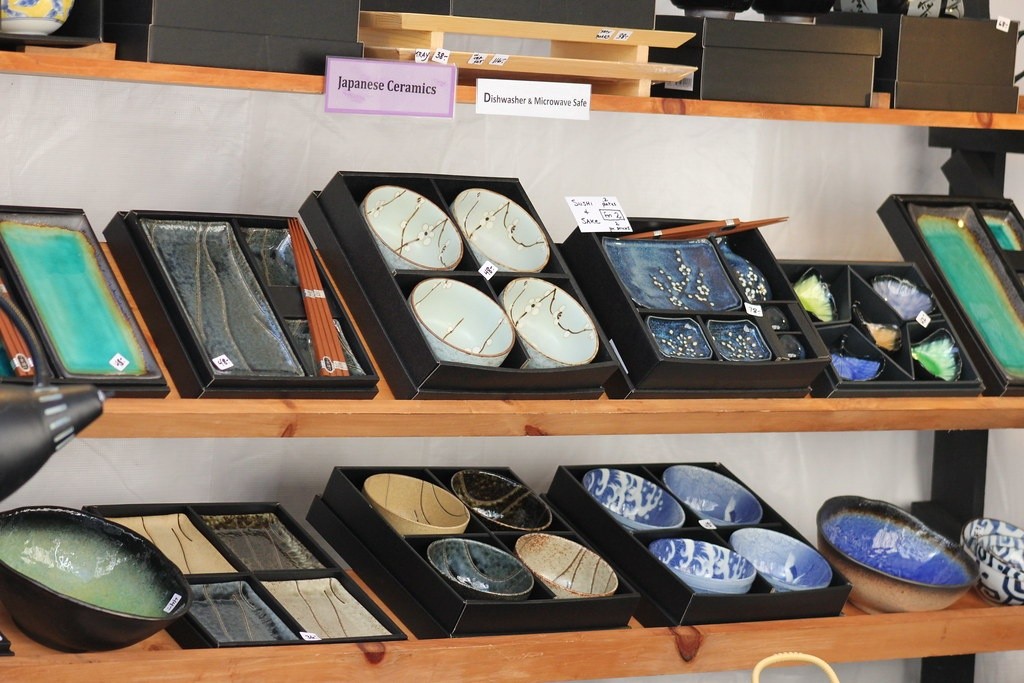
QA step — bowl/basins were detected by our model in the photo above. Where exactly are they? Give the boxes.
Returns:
[452,471,552,531]
[450,189,550,272]
[407,278,514,368]
[0,0,74,36]
[515,533,618,597]
[583,469,685,532]
[498,276,599,367]
[428,539,534,600]
[817,496,979,616]
[731,527,832,592]
[649,538,756,595]
[365,474,469,534]
[0,504,193,650]
[962,517,1024,608]
[665,465,763,527]
[359,185,462,271]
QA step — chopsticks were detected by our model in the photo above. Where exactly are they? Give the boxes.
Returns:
[624,216,788,233]
[0,278,47,377]
[286,217,350,377]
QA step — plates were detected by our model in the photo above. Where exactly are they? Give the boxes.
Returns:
[647,315,713,361]
[981,209,1024,251]
[708,320,772,363]
[188,583,299,645]
[601,236,742,312]
[245,226,302,287]
[199,512,329,569]
[284,318,364,377]
[0,215,163,380]
[105,514,237,576]
[144,220,303,378]
[910,205,1024,379]
[264,578,391,639]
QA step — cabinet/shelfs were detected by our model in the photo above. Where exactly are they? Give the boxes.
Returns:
[0,44,1024,683]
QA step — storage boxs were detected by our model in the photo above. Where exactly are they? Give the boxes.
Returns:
[0,0,1022,653]
